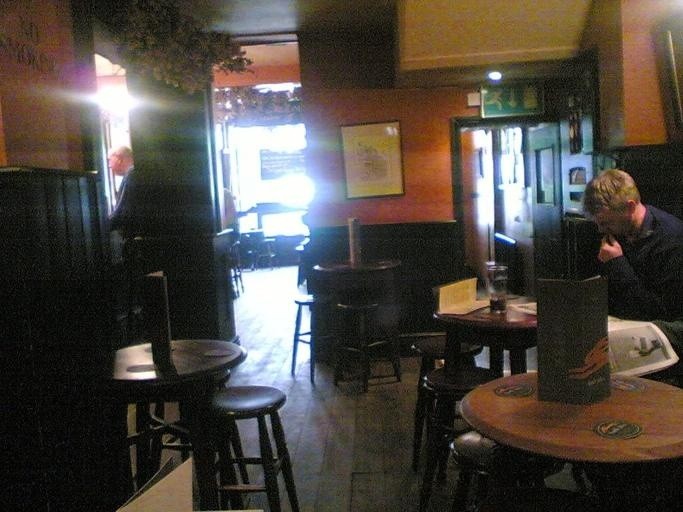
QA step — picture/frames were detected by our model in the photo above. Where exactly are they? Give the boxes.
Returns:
[339,119,406,200]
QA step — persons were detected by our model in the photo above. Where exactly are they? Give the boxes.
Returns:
[578,167,682,389]
[103,144,145,315]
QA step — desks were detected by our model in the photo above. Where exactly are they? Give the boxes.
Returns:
[111,340,244,510]
[433,295,538,431]
[241,230,264,271]
[459,372,683,512]
[311,256,404,382]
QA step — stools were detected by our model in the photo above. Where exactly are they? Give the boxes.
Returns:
[412,334,482,439]
[334,303,401,392]
[213,386,300,512]
[451,433,502,512]
[292,294,336,382]
[256,239,281,271]
[295,244,307,289]
[420,365,502,512]
[228,257,244,297]
[152,369,250,484]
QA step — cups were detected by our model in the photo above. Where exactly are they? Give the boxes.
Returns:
[486,265,508,315]
